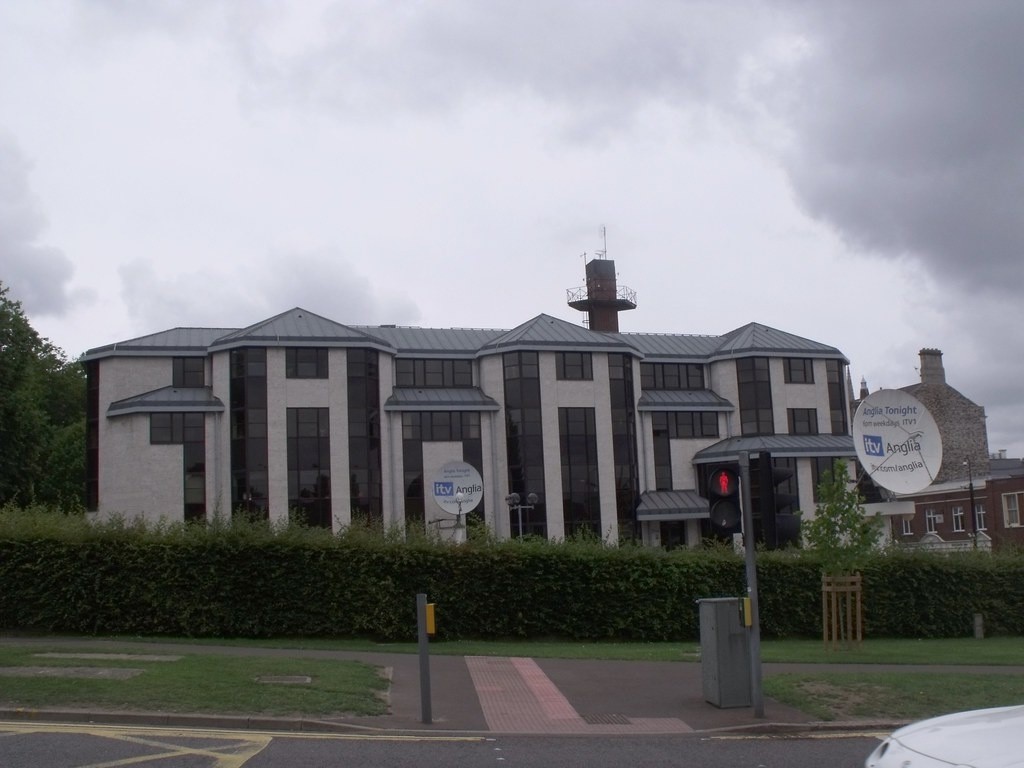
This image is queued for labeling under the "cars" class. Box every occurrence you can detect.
[866,704,1024,768]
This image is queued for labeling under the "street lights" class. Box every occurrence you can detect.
[963,454,977,547]
[505,492,538,540]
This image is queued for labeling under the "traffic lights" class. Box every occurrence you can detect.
[702,461,742,535]
[757,451,802,551]
